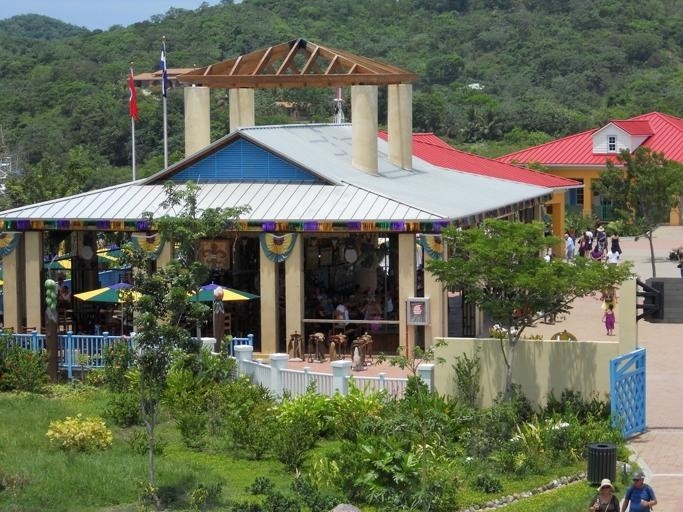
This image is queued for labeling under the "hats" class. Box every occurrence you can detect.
[598,478,615,493]
[632,472,644,480]
[597,226,604,232]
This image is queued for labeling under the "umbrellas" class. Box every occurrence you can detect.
[183,281,262,337]
[72,280,150,336]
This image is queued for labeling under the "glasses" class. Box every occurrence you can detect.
[602,486,609,489]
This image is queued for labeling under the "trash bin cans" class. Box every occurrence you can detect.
[587,443,617,487]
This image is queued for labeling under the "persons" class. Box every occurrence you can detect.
[620,471,657,512]
[599,304,614,336]
[304,267,396,338]
[585,479,620,512]
[564,233,574,263]
[598,286,617,316]
[57,284,71,308]
[577,215,622,266]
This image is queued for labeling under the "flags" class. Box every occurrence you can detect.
[159,38,171,98]
[128,72,140,122]
